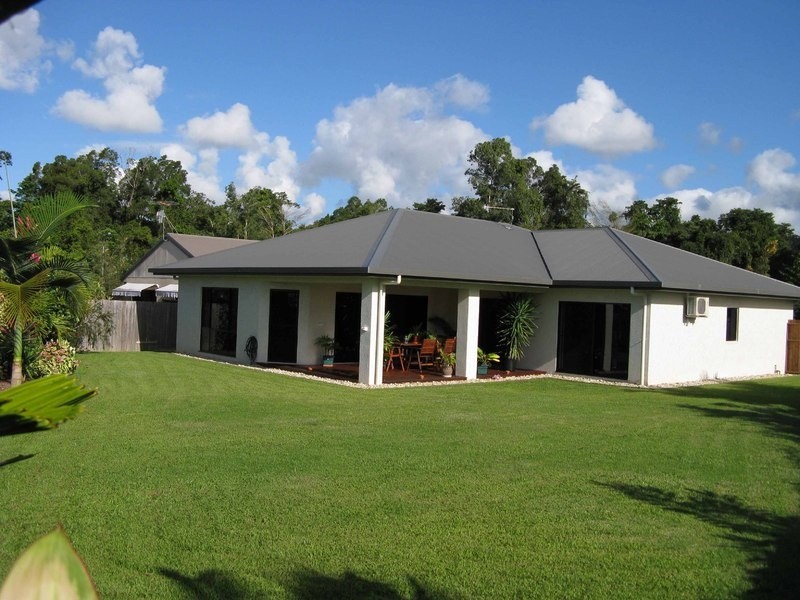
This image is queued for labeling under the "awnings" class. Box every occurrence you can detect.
[112,283,160,297]
[155,284,178,298]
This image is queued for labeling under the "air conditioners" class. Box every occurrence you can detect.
[685,295,710,318]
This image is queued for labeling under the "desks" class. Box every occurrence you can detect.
[392,342,422,370]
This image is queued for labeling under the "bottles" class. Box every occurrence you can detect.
[404,336,408,344]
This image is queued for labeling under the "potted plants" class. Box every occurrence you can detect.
[314,335,335,368]
[495,295,541,372]
[440,353,456,378]
[477,346,501,375]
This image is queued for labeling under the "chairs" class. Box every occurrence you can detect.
[387,345,406,372]
[415,338,437,374]
[438,339,456,359]
